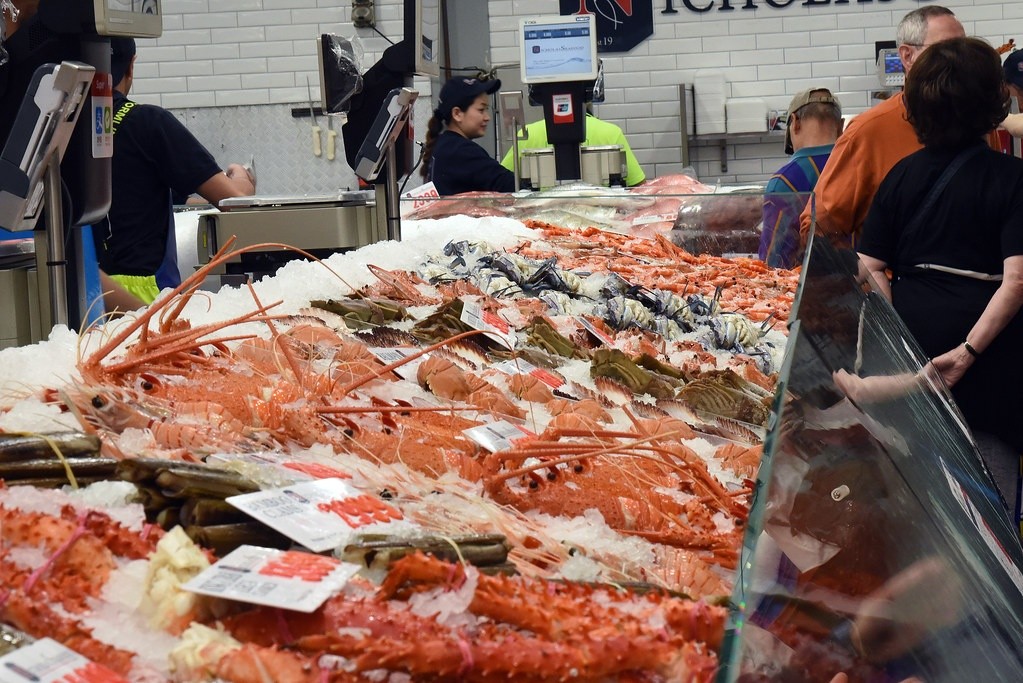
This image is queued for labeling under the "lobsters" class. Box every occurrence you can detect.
[59,230,764,575]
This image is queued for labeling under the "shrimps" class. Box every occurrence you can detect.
[357,217,802,390]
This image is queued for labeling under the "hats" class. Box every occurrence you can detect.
[784,87,842,155]
[1002,47,1023,88]
[438,76,501,120]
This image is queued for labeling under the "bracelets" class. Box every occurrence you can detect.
[964,340,978,358]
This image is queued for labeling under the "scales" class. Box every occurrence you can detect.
[193,86,420,275]
[0,60,97,351]
[517,13,627,191]
[72,0,163,228]
[875,47,906,93]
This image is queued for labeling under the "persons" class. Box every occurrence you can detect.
[90,36,258,320]
[799,5,1023,541]
[420,76,540,196]
[500,101,646,190]
[758,87,845,269]
[739,217,1022,683]
